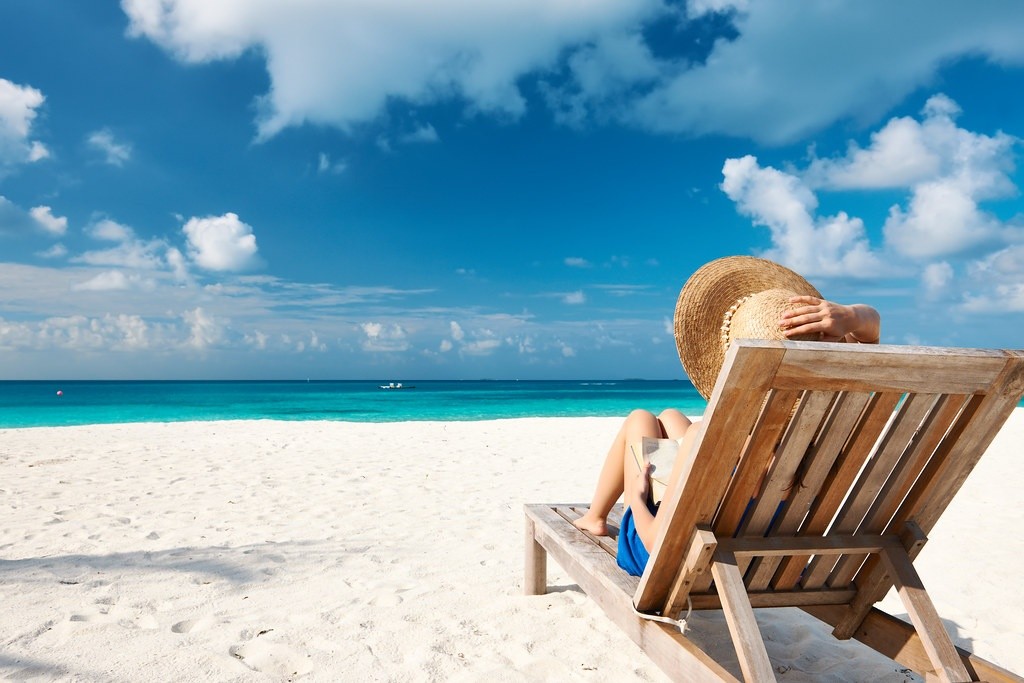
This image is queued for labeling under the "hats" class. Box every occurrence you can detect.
[674,255,849,446]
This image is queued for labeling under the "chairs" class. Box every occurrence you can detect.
[523,339,1024,682]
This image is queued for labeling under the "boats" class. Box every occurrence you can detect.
[377,384,416,389]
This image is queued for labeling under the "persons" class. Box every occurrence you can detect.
[570,295,881,578]
